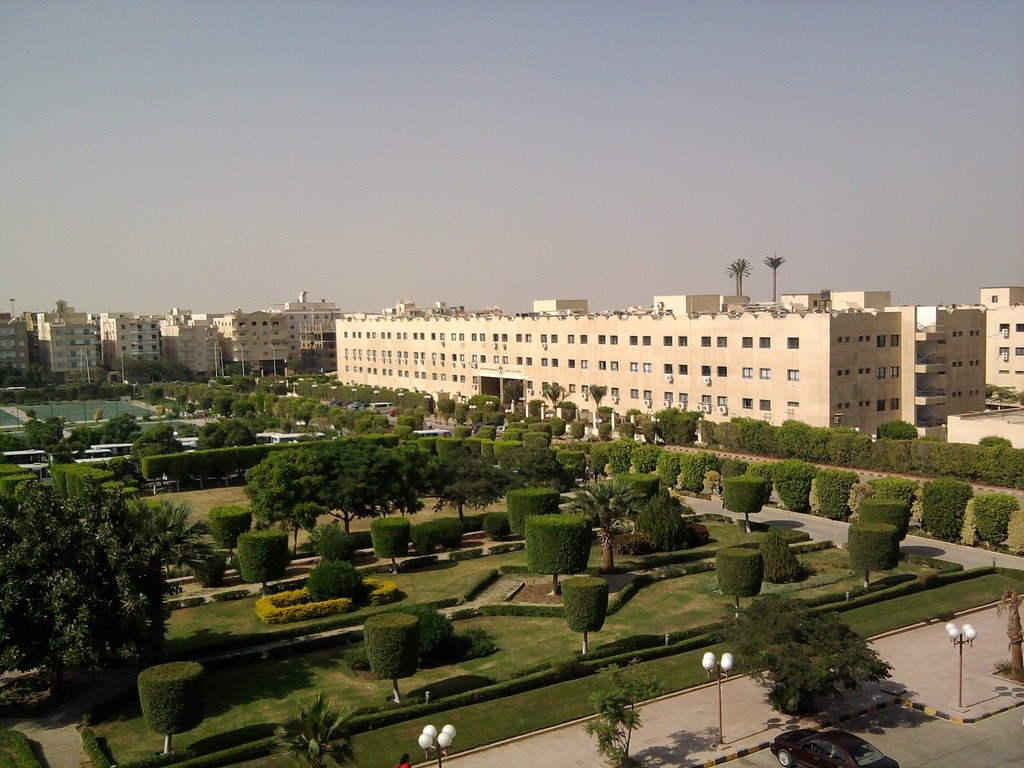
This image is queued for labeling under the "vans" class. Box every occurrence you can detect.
[496,426,508,436]
[370,403,395,414]
[7,386,26,390]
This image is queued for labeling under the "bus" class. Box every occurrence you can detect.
[3,450,51,471]
[72,449,116,462]
[414,428,453,438]
[91,444,135,459]
[271,431,326,442]
[176,437,198,452]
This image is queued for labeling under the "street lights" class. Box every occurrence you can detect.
[268,343,277,383]
[418,725,456,768]
[237,341,245,379]
[214,341,224,379]
[702,652,733,744]
[946,623,976,706]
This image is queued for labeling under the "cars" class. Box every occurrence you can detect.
[768,727,900,768]
[330,401,369,411]
